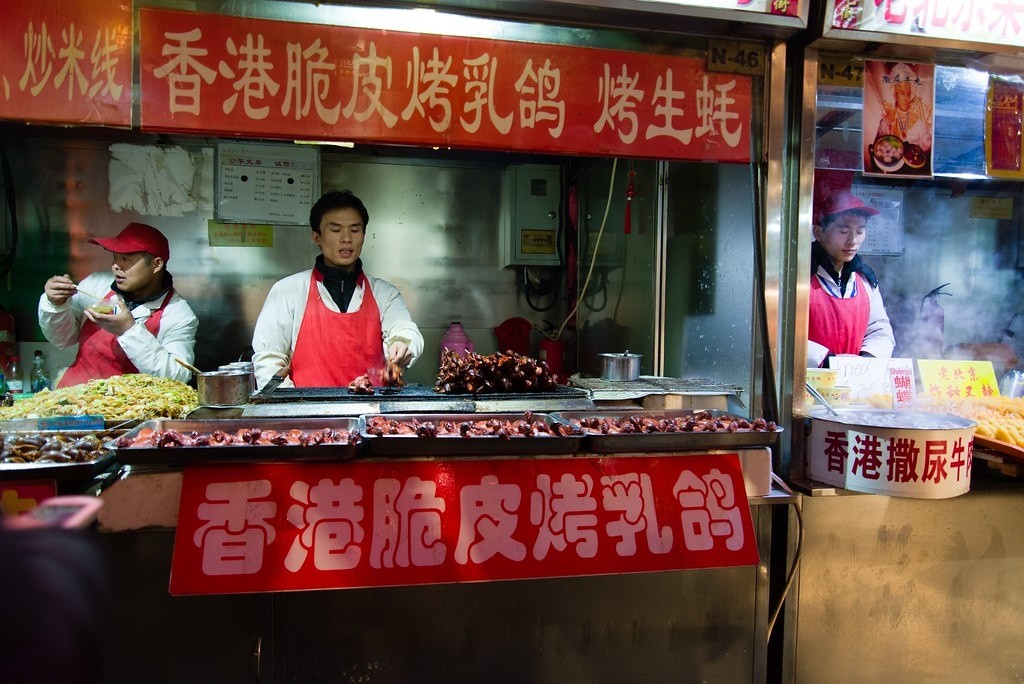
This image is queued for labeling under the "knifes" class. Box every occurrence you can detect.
[261,367,291,394]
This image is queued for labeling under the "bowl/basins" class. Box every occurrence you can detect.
[805,409,978,499]
[805,367,835,403]
[79,299,118,314]
[197,372,251,405]
[600,353,642,382]
[815,387,852,405]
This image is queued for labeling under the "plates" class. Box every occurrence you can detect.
[903,155,926,169]
[874,157,904,172]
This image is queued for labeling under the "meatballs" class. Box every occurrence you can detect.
[876,142,901,163]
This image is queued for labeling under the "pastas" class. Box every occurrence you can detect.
[0,373,199,419]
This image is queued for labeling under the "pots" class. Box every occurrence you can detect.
[869,135,909,165]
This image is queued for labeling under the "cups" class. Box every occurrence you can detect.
[230,362,255,394]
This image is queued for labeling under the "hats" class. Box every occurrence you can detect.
[812,190,881,224]
[884,61,920,91]
[86,222,169,264]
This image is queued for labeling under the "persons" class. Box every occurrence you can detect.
[251,189,424,386]
[806,188,896,368]
[38,223,198,388]
[875,80,933,152]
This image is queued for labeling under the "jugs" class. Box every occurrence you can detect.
[441,322,474,362]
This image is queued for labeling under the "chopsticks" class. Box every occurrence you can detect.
[73,283,103,299]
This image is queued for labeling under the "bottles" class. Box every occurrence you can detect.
[6,357,23,394]
[29,350,51,394]
[0,366,5,394]
